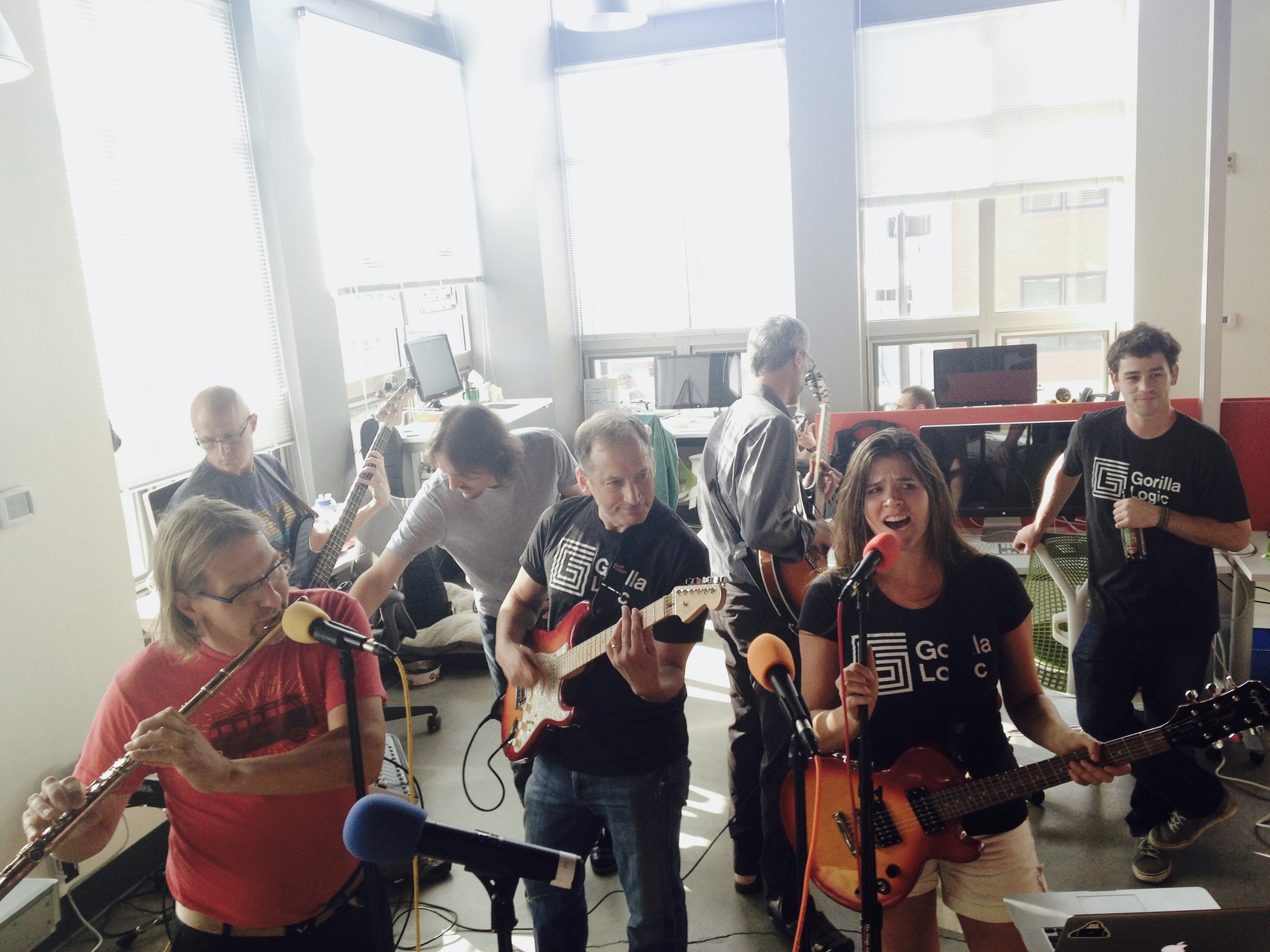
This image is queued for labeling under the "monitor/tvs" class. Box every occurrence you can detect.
[920,419,1087,543]
[404,334,464,411]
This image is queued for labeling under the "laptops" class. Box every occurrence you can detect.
[1002,886,1270,952]
[934,342,1037,408]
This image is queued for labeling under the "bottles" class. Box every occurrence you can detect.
[1118,489,1147,563]
[314,493,336,519]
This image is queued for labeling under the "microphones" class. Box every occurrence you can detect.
[839,532,900,603]
[281,602,397,660]
[747,633,821,757]
[343,794,585,890]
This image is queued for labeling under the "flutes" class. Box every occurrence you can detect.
[0,594,309,904]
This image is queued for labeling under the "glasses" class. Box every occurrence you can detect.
[193,416,249,449]
[802,351,816,374]
[196,544,293,606]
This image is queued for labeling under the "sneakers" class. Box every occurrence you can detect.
[1132,835,1173,883]
[1148,794,1241,850]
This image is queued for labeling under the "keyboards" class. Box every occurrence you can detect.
[968,542,1026,556]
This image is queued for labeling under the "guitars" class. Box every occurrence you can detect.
[288,374,419,590]
[778,676,1268,915]
[499,572,733,764]
[758,368,837,631]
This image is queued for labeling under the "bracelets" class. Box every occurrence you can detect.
[1156,507,1169,530]
[806,445,816,453]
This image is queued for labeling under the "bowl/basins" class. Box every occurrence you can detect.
[398,659,442,686]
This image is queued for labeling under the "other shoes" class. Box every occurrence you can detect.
[590,826,619,876]
[778,911,855,952]
[734,879,765,895]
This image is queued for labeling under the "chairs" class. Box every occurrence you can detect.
[632,415,700,529]
[367,589,438,733]
[1023,533,1091,698]
[1012,440,1068,508]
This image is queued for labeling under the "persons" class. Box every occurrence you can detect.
[346,400,616,874]
[797,428,1131,952]
[894,386,968,511]
[992,421,1086,506]
[697,314,856,952]
[1012,321,1252,882]
[164,387,390,589]
[495,408,711,951]
[796,420,817,458]
[22,491,393,952]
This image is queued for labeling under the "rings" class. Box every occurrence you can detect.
[611,644,621,647]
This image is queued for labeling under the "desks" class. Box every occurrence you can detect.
[632,407,729,447]
[962,528,1270,773]
[394,397,554,497]
[138,545,355,634]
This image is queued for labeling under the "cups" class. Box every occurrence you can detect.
[462,388,480,403]
